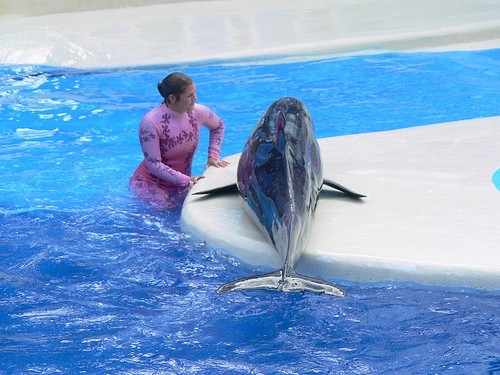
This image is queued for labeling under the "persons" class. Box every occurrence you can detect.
[127,72,232,210]
